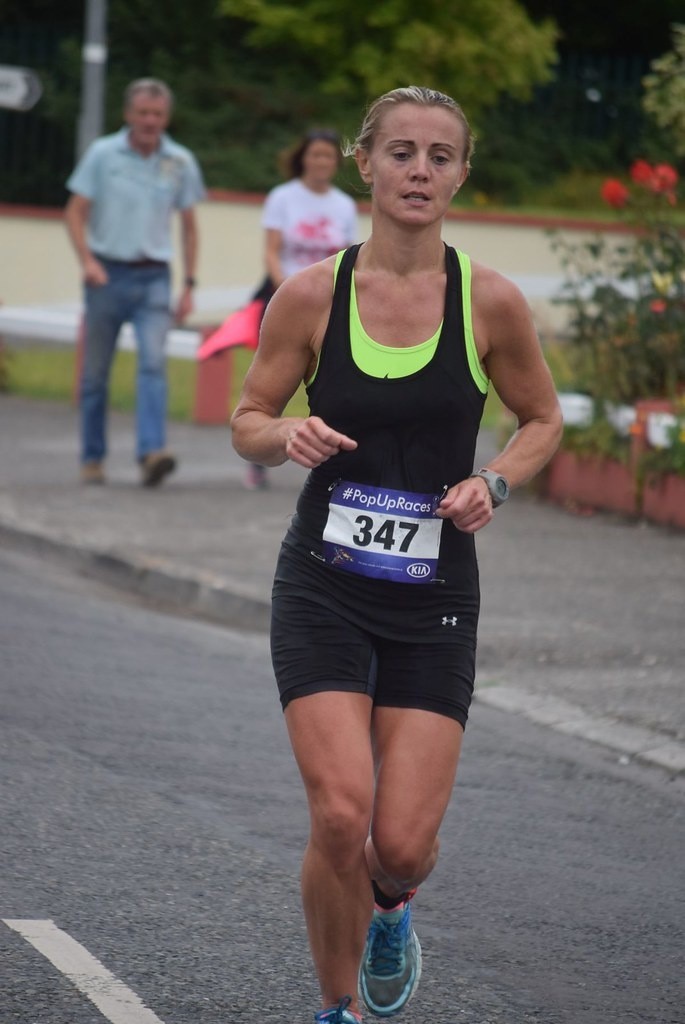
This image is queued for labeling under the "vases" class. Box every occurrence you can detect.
[543,392,684,527]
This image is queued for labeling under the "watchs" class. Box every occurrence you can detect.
[471,468,509,510]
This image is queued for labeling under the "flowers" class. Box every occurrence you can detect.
[548,157,685,471]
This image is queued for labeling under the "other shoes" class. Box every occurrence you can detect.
[140,452,176,487]
[79,460,103,483]
[243,459,266,490]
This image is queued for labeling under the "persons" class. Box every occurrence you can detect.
[241,132,361,492]
[232,87,563,1024]
[62,79,204,487]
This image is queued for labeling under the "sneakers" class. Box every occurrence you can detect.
[360,885,422,1017]
[314,995,361,1024]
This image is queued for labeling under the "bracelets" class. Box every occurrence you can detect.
[184,279,195,286]
